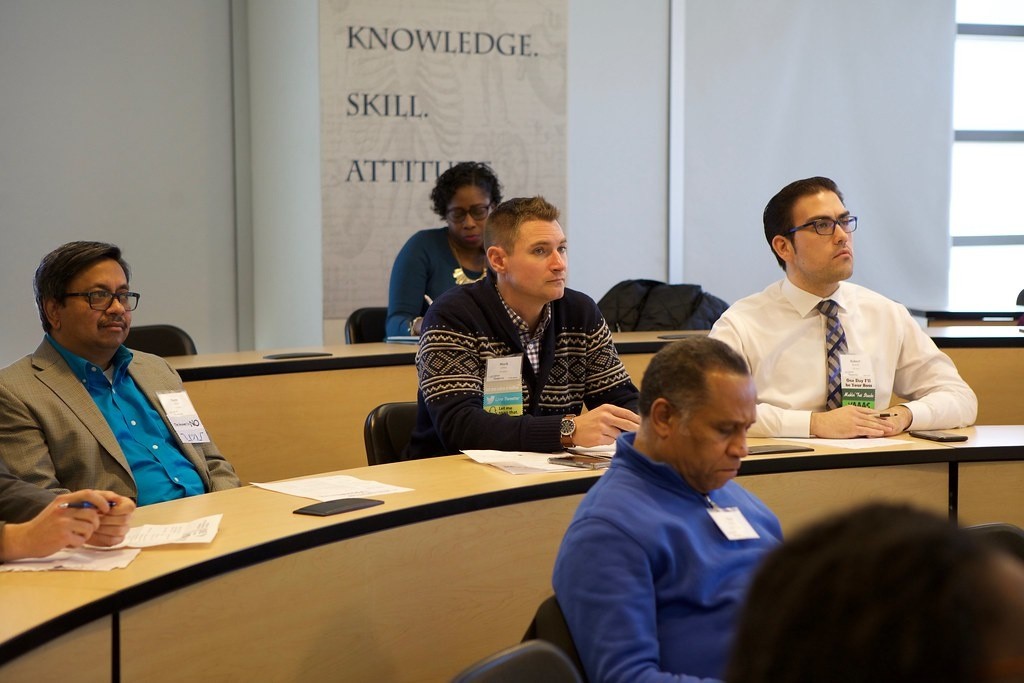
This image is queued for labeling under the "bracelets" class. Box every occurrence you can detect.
[410,317,422,335]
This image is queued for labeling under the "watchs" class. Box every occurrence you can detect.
[561,414,576,447]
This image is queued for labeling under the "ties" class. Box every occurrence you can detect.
[817,300,848,411]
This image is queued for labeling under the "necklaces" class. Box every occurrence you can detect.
[452,246,488,285]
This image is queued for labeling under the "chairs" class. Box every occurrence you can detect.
[120,325,197,358]
[365,402,420,466]
[344,307,388,345]
[451,594,590,683]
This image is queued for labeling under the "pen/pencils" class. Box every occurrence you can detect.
[58,501,115,509]
[870,414,898,417]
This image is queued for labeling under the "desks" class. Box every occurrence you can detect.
[0,316,1023,683]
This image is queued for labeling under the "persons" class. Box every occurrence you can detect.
[709,176,978,439]
[385,161,503,337]
[553,335,785,682]
[729,504,1024,683]
[0,460,135,561]
[0,240,239,506]
[416,196,640,460]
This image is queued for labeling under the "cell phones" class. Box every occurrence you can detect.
[909,431,968,442]
[548,455,612,470]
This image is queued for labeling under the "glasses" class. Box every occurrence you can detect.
[782,216,858,235]
[55,290,140,311]
[443,199,494,223]
